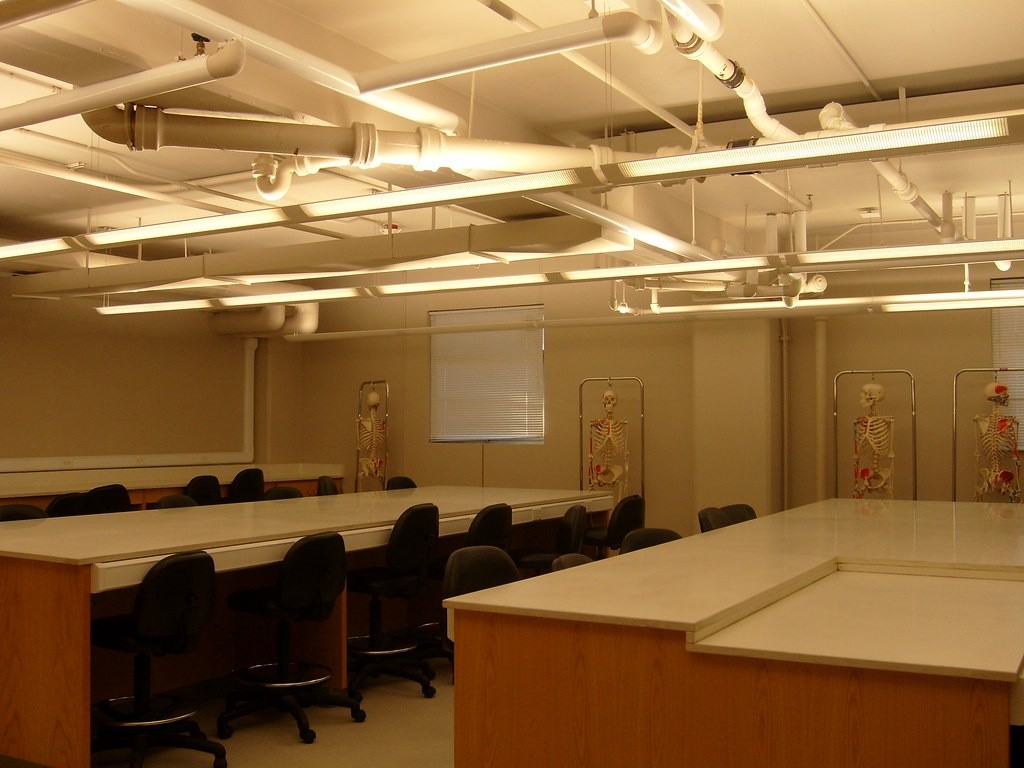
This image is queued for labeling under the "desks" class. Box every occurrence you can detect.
[0,486,614,767]
[0,464,347,512]
[443,498,1024,768]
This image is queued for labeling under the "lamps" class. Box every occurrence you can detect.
[0,109,1023,314]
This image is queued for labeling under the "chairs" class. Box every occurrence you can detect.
[89,549,226,767]
[416,495,756,684]
[216,532,367,743]
[0,472,419,522]
[348,502,456,698]
